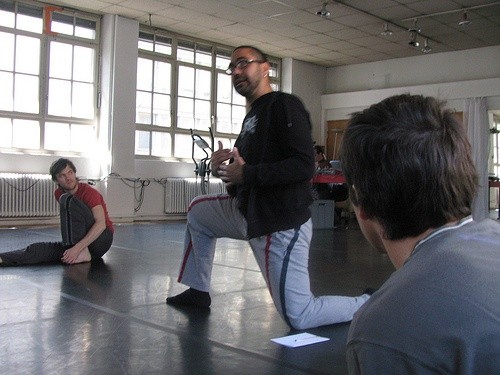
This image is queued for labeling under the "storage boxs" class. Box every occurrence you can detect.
[310,199,334,229]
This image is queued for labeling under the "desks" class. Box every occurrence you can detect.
[488,180,500,220]
[309,175,350,230]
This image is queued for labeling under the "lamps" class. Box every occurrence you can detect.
[315,0,331,16]
[380,22,393,36]
[422,37,432,52]
[457,9,472,27]
[407,19,420,46]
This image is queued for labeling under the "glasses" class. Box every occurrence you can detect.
[225,60,266,75]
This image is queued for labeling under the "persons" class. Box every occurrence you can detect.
[0,158,114,268]
[166,45,375,332]
[344,94,500,375]
[312,144,332,199]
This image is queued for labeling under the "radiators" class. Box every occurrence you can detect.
[165,178,226,214]
[0,171,60,217]
